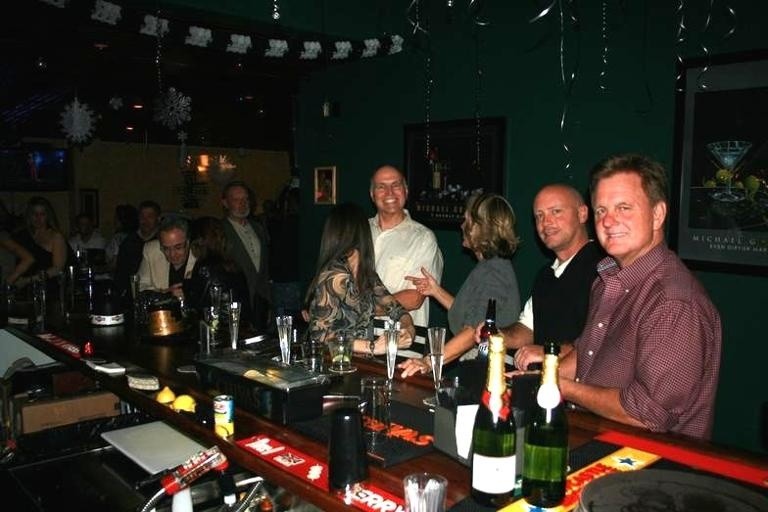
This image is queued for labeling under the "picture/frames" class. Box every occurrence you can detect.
[311,164,338,205]
[403,114,508,234]
[665,49,768,277]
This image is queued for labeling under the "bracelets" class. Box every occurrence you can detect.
[369,339,375,356]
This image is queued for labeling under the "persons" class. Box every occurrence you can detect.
[136,216,251,327]
[366,161,445,329]
[67,200,162,300]
[398,189,521,379]
[475,183,604,376]
[221,182,323,327]
[302,198,418,354]
[1,197,69,297]
[536,150,723,444]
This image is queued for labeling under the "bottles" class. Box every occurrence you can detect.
[467,335,516,509]
[521,341,569,509]
[413,201,464,219]
[475,296,498,357]
[213,458,292,512]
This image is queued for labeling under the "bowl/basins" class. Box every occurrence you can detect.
[577,469,768,511]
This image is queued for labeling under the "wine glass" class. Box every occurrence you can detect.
[704,139,752,203]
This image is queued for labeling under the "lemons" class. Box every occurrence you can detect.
[173,394,196,412]
[156,385,175,404]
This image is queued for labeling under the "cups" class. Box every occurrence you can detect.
[432,160,450,190]
[300,338,325,374]
[325,333,352,369]
[381,330,404,383]
[359,377,392,447]
[327,406,366,491]
[274,314,293,365]
[25,262,140,330]
[201,286,240,350]
[400,471,448,511]
[426,324,446,401]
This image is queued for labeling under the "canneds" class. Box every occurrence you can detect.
[212,393,236,439]
